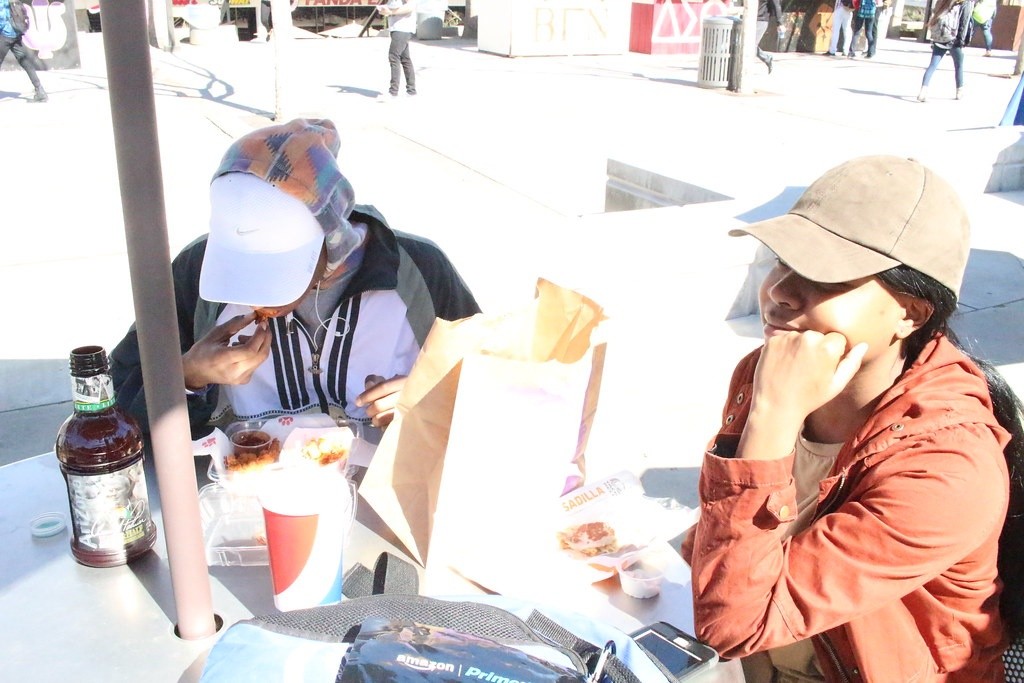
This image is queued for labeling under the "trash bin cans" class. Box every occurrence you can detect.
[697,16,740,89]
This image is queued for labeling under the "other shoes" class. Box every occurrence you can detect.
[865,51,874,60]
[376,92,398,103]
[847,53,855,57]
[983,50,991,57]
[766,56,774,74]
[27,93,48,104]
[822,51,836,57]
[399,91,417,101]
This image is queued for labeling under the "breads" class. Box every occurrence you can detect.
[570,522,614,553]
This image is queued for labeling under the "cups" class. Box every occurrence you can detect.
[256,472,350,612]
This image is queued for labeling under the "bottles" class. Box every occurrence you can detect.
[56,345,157,568]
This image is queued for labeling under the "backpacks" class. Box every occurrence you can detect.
[8,0,30,34]
[930,1,970,44]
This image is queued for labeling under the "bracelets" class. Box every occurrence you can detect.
[389,10,392,16]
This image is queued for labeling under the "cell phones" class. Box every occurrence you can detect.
[627,621,719,682]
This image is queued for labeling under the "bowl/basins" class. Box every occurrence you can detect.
[230,429,271,457]
[207,418,364,482]
[612,549,666,598]
[197,480,358,566]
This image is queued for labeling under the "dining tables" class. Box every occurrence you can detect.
[1,417,747,682]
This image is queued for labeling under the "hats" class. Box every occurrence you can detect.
[198,173,325,306]
[728,157,971,309]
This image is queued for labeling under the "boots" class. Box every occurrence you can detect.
[917,86,926,102]
[956,87,963,101]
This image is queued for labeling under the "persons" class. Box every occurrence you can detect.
[0,0,48,102]
[917,0,996,102]
[681,154,1023,683]
[261,0,296,41]
[822,0,891,59]
[379,0,417,102]
[757,0,786,74]
[106,120,483,440]
